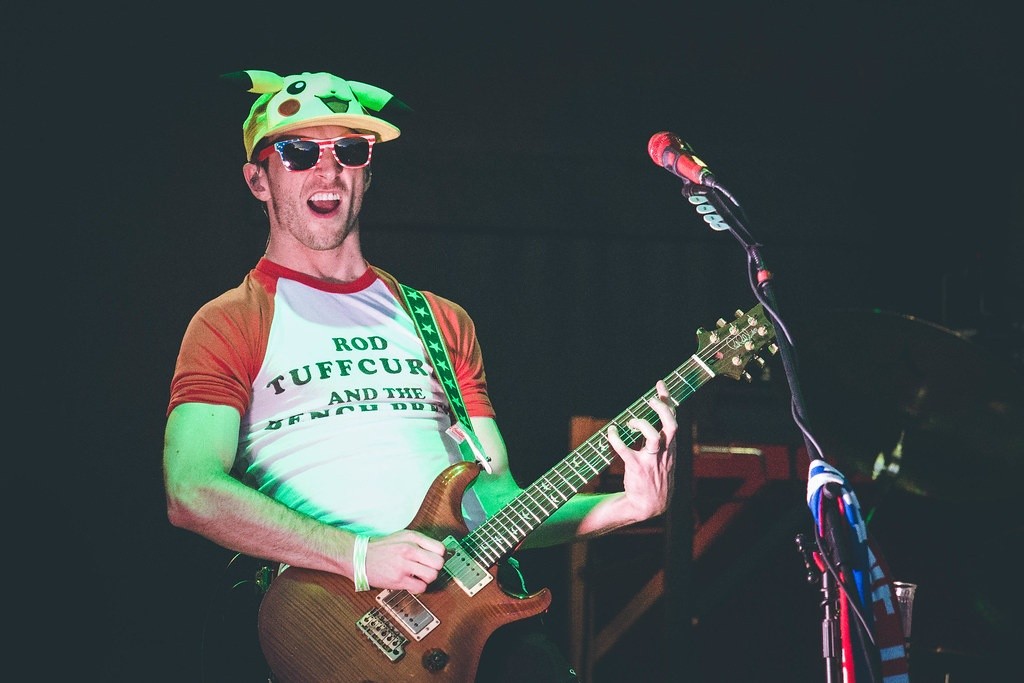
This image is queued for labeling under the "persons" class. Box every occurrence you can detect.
[162,69,680,683]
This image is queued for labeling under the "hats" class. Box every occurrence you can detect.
[242,70,400,164]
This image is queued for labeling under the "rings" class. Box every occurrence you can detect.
[644,446,661,455]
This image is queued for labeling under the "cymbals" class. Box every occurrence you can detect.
[792,306,1004,437]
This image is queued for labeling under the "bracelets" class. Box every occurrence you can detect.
[353,533,371,592]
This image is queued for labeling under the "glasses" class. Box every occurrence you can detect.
[258,133,377,171]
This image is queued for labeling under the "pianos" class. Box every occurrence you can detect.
[571,415,842,478]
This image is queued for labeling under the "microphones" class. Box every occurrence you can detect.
[648,131,716,187]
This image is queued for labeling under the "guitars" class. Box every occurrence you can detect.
[256,300,783,683]
